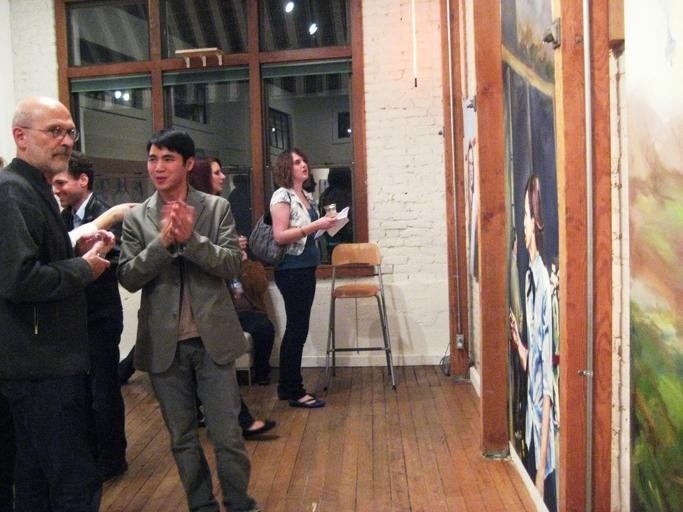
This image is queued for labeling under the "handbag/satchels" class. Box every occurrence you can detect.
[249,208,291,264]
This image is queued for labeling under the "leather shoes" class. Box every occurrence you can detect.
[289,393,325,408]
[243,419,277,436]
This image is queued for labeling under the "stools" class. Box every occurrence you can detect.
[229,331,254,391]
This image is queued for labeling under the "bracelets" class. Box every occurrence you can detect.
[300,227,307,239]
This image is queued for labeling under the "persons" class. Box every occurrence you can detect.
[53,152,129,482]
[508,175,557,512]
[118,128,252,509]
[184,157,275,439]
[268,149,337,409]
[228,251,275,385]
[462,139,480,285]
[0,96,112,511]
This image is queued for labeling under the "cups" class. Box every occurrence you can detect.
[323,203,336,219]
[87,235,108,258]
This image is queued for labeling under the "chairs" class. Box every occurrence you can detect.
[324,243,397,391]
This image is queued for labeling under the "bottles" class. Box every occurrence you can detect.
[230,276,244,298]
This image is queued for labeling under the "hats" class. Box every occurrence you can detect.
[239,259,268,310]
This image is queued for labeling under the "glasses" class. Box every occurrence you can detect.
[20,125,80,141]
